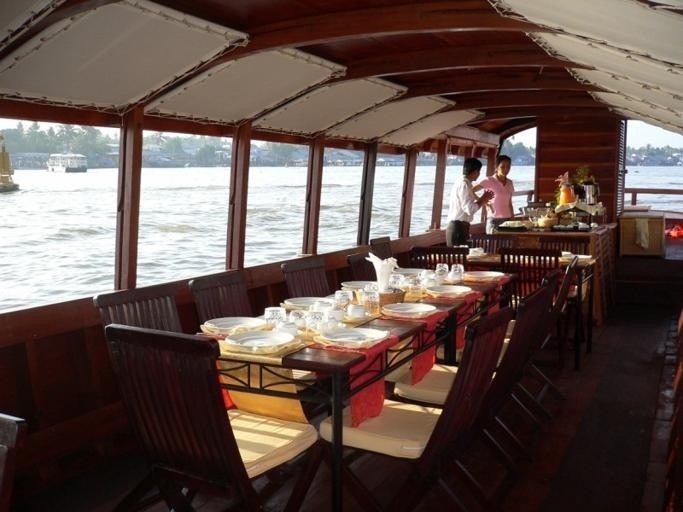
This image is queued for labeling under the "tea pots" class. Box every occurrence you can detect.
[559,182,576,203]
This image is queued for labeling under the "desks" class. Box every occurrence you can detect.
[493,222,618,329]
[413,246,595,350]
[197,268,508,507]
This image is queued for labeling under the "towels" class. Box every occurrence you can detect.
[635,218,649,249]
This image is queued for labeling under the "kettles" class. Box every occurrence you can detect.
[583,180,599,205]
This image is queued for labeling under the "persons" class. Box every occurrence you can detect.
[472,155,514,235]
[445,157,495,248]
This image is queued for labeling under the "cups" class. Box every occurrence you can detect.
[390,264,464,298]
[335,290,378,319]
[264,307,323,335]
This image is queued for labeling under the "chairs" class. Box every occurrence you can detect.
[539,234,595,352]
[295,309,516,511]
[0,412,27,512]
[372,238,393,261]
[347,249,380,283]
[105,322,344,511]
[409,245,431,268]
[98,287,181,335]
[471,233,516,253]
[498,243,566,300]
[188,270,255,322]
[396,256,579,471]
[281,254,331,299]
[428,245,469,272]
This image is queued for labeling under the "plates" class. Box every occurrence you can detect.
[205,317,264,330]
[322,328,387,343]
[464,270,502,278]
[428,285,471,295]
[226,331,294,349]
[383,302,434,314]
[341,281,378,288]
[284,297,332,307]
